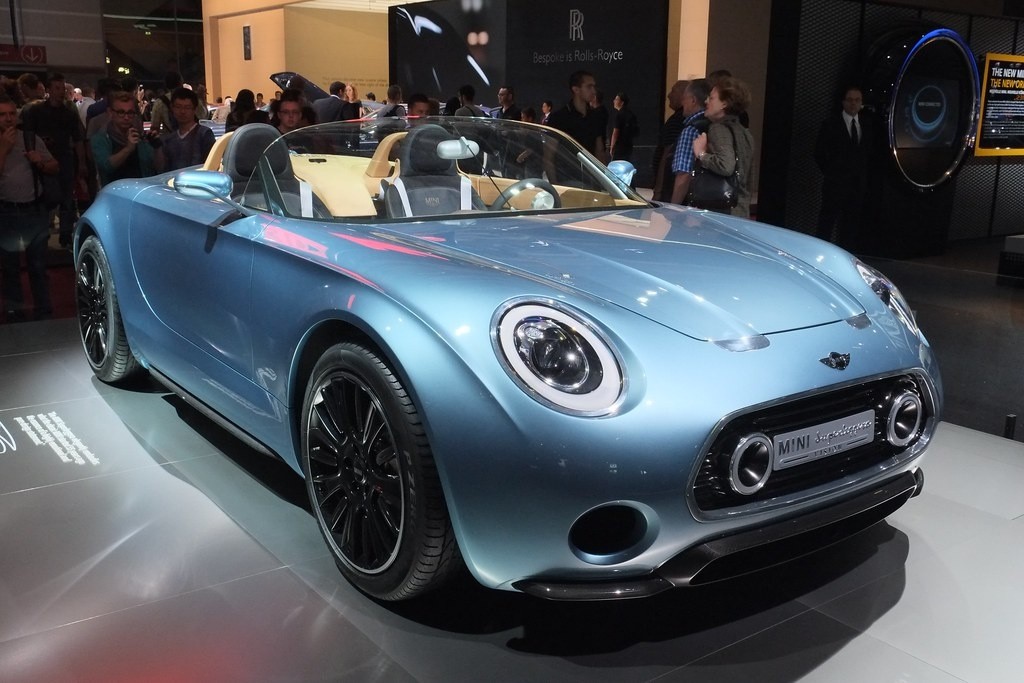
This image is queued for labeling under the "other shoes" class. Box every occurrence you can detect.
[33,300,54,320]
[58,232,73,247]
[3,299,24,322]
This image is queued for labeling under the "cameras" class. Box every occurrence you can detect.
[133,131,149,140]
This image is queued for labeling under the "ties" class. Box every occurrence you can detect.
[850,119,858,150]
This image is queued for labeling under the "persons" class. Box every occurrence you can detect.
[539,100,551,129]
[811,87,875,253]
[161,88,215,170]
[224,87,270,132]
[0,92,60,319]
[588,89,608,141]
[495,85,523,179]
[0,71,387,234]
[375,88,407,144]
[19,71,87,253]
[406,90,464,134]
[451,85,485,176]
[651,67,756,220]
[88,89,165,190]
[609,92,634,162]
[297,106,332,144]
[273,88,314,153]
[516,108,544,179]
[542,70,605,190]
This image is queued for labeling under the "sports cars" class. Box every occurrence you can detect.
[74,70,943,603]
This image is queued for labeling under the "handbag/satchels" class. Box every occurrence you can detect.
[39,177,64,209]
[682,121,739,215]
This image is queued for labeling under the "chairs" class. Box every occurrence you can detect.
[385,124,488,219]
[222,122,334,218]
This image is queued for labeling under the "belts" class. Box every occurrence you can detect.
[2,201,33,208]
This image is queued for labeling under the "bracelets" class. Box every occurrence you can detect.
[698,152,706,159]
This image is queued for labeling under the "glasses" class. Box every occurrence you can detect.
[110,107,134,115]
[278,108,301,116]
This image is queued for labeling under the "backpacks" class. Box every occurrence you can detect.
[375,105,403,142]
